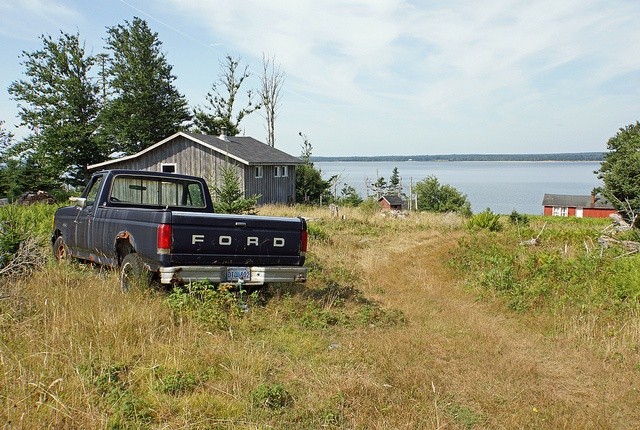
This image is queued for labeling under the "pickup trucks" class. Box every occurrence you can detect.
[51,169,308,297]
